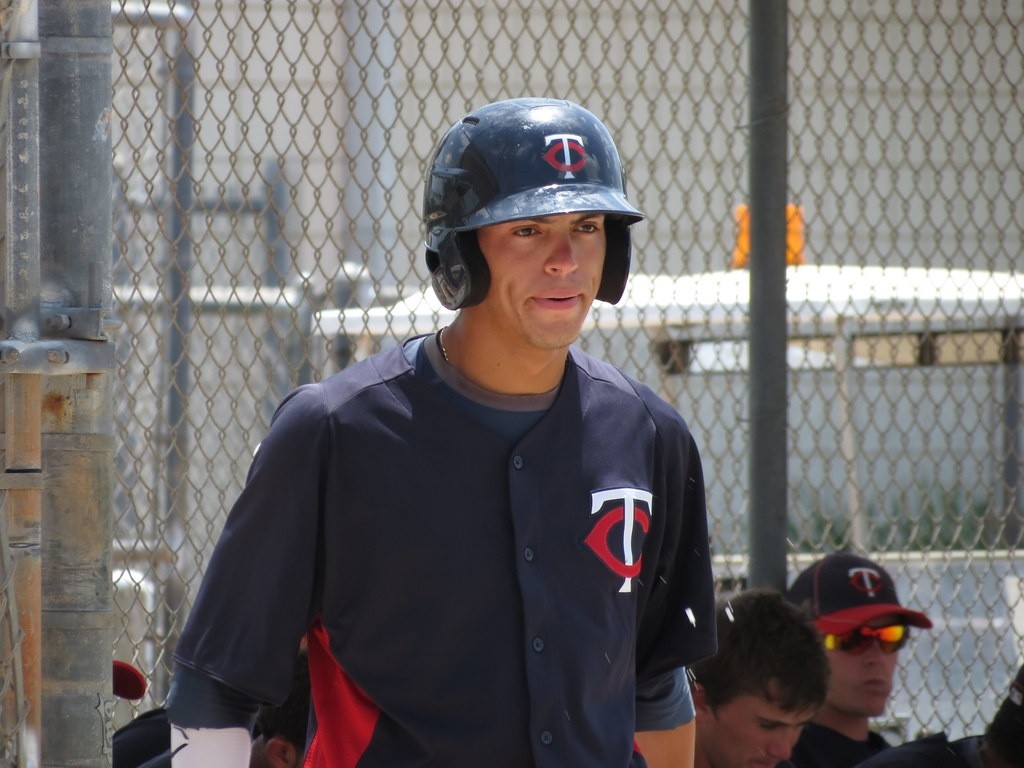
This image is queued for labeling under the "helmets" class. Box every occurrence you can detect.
[423,98,645,310]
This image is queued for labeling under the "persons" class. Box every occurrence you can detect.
[630,550,1024,768]
[164,96,715,767]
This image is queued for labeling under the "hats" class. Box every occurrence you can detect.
[784,553,933,637]
[113,659,147,700]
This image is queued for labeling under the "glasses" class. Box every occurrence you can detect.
[821,623,909,654]
